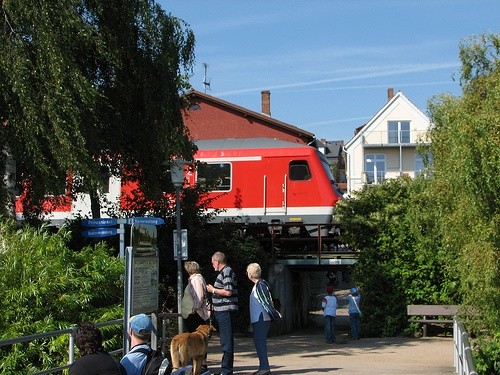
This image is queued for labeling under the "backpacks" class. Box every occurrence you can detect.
[132,348,172,375]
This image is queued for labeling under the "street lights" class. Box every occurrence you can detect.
[171,166,184,338]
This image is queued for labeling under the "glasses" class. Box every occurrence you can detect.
[246,271,248,275]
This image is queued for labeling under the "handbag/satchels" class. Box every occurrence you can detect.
[272,298,281,320]
[359,311,364,321]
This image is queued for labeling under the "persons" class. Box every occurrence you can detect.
[346,287,362,341]
[206,252,241,375]
[67,323,128,375]
[181,261,210,370]
[247,263,283,375]
[120,313,164,375]
[321,287,337,344]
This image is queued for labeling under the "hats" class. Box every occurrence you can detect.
[129,313,152,335]
[350,287,358,293]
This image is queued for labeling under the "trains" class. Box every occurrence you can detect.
[15,138,339,235]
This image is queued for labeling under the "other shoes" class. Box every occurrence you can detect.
[350,335,359,340]
[214,368,223,375]
[326,338,336,344]
[199,364,210,375]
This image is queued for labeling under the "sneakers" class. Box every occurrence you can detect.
[255,369,270,375]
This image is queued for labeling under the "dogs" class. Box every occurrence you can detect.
[170,325,216,375]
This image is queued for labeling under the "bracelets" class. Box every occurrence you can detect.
[213,288,215,294]
